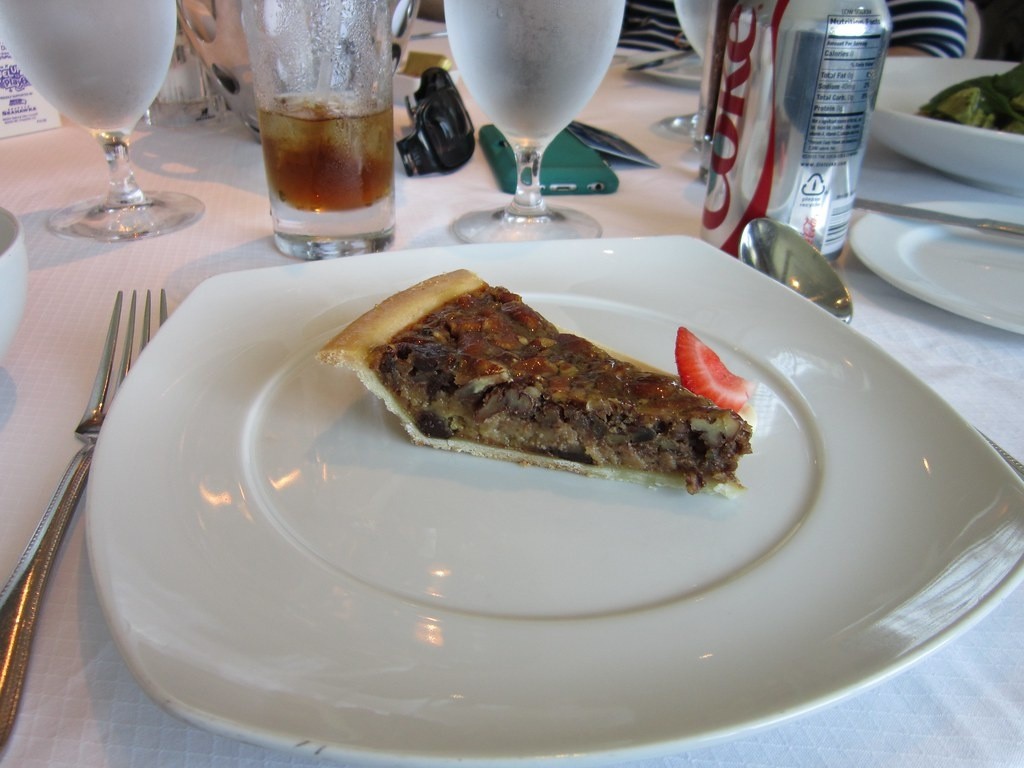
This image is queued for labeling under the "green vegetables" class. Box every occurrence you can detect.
[919,61,1024,138]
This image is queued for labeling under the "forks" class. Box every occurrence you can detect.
[0,289,166,753]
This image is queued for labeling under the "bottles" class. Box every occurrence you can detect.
[699,1,739,184]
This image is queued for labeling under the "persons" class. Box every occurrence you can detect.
[617,0,966,59]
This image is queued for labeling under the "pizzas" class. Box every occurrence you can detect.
[317,269,754,499]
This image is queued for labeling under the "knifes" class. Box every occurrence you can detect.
[852,197,1023,240]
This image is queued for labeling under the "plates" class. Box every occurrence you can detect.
[849,211,1023,335]
[83,235,1023,762]
[631,49,707,87]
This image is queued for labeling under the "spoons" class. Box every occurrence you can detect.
[736,219,1024,483]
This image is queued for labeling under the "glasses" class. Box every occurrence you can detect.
[396,67,475,176]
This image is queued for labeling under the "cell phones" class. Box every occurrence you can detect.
[479,124,620,196]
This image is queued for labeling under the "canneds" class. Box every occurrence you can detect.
[700,0,891,273]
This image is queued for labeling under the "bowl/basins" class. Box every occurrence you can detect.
[177,0,420,143]
[869,56,1024,198]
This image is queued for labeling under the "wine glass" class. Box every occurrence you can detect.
[660,0,724,142]
[1,0,205,242]
[444,0,626,241]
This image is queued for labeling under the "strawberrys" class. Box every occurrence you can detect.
[675,325,753,416]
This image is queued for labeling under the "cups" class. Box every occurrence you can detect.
[0,208,28,366]
[241,1,396,260]
